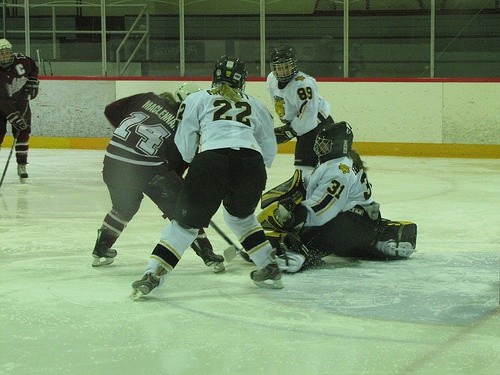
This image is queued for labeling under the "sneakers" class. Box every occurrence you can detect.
[129,271,158,304]
[277,251,306,273]
[92,229,118,267]
[17,164,29,181]
[374,238,418,258]
[202,247,228,273]
[250,263,284,290]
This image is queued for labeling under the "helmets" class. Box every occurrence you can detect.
[0,39,13,65]
[270,45,300,85]
[314,121,354,163]
[177,82,202,103]
[212,54,249,88]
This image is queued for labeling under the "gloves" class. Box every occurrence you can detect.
[274,125,297,145]
[7,111,30,133]
[25,78,40,100]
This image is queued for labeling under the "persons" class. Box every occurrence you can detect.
[92,82,225,273]
[131,55,283,299]
[0,39,40,183]
[266,45,334,184]
[277,121,416,274]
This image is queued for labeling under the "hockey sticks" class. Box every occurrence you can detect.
[209,220,256,265]
[0,93,30,189]
